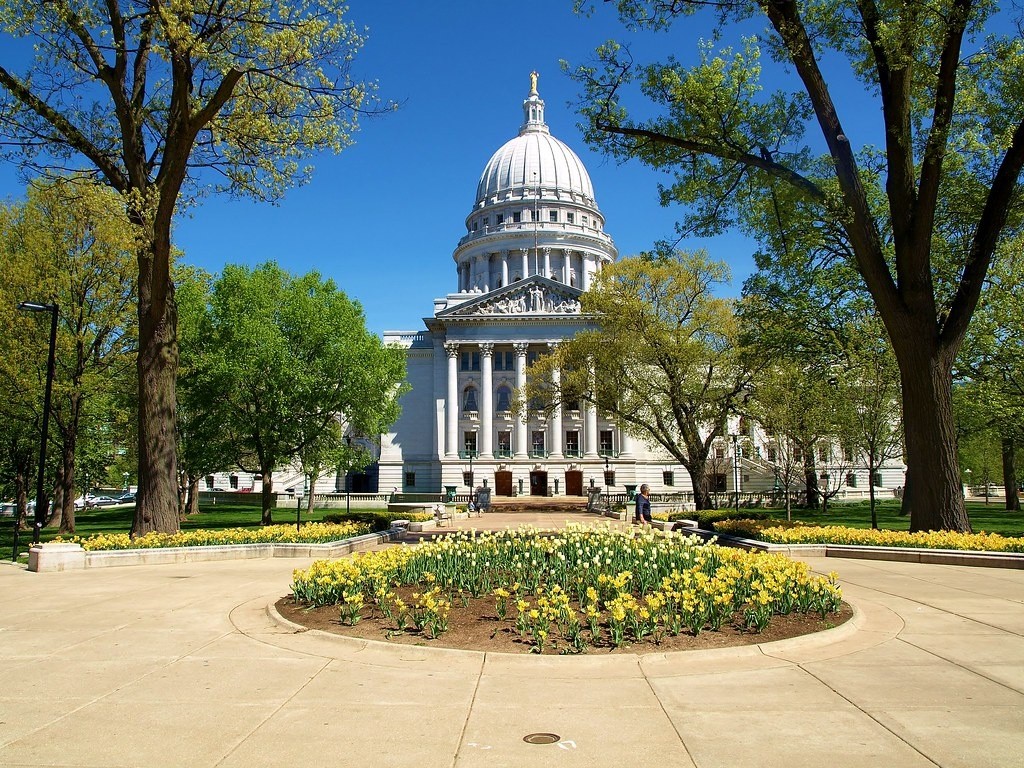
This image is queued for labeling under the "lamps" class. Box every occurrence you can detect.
[570,462,578,470]
[499,463,506,470]
[534,463,542,471]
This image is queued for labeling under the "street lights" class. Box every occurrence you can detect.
[16,301,60,545]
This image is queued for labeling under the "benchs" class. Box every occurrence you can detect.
[435,507,453,527]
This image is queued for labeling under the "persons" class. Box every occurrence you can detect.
[470,286,582,315]
[394,486,398,493]
[27,504,32,513]
[82,501,91,511]
[799,497,806,504]
[468,500,484,518]
[898,486,905,496]
[636,484,653,533]
[530,71,539,91]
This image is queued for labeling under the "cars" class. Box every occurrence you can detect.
[207,488,225,492]
[234,487,252,493]
[118,492,138,504]
[73,495,96,509]
[86,496,123,507]
